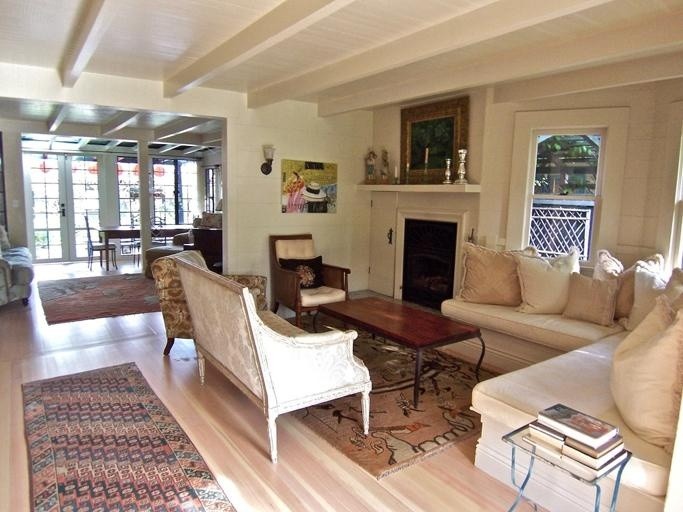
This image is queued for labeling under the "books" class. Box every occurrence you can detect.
[522,403,628,478]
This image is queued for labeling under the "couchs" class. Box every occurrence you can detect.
[440,248,683,512]
[0,226,35,310]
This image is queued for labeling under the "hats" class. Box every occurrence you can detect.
[304,182,326,199]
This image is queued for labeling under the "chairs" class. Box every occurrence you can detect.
[150,249,268,357]
[268,232,352,326]
[79,196,224,279]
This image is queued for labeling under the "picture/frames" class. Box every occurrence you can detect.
[396,94,471,184]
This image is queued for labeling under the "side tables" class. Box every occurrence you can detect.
[501,417,633,512]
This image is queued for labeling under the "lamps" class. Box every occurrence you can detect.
[261,146,275,176]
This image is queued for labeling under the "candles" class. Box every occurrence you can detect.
[424,149,429,163]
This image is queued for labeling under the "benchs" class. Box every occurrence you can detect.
[174,255,372,462]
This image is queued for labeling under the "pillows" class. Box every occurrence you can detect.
[592,249,665,320]
[512,249,581,314]
[610,295,681,453]
[617,266,669,331]
[458,247,541,306]
[279,255,324,291]
[562,272,618,328]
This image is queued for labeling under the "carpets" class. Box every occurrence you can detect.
[19,361,235,511]
[36,275,163,325]
[294,330,496,484]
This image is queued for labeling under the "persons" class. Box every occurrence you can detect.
[366,150,377,181]
[284,171,328,213]
